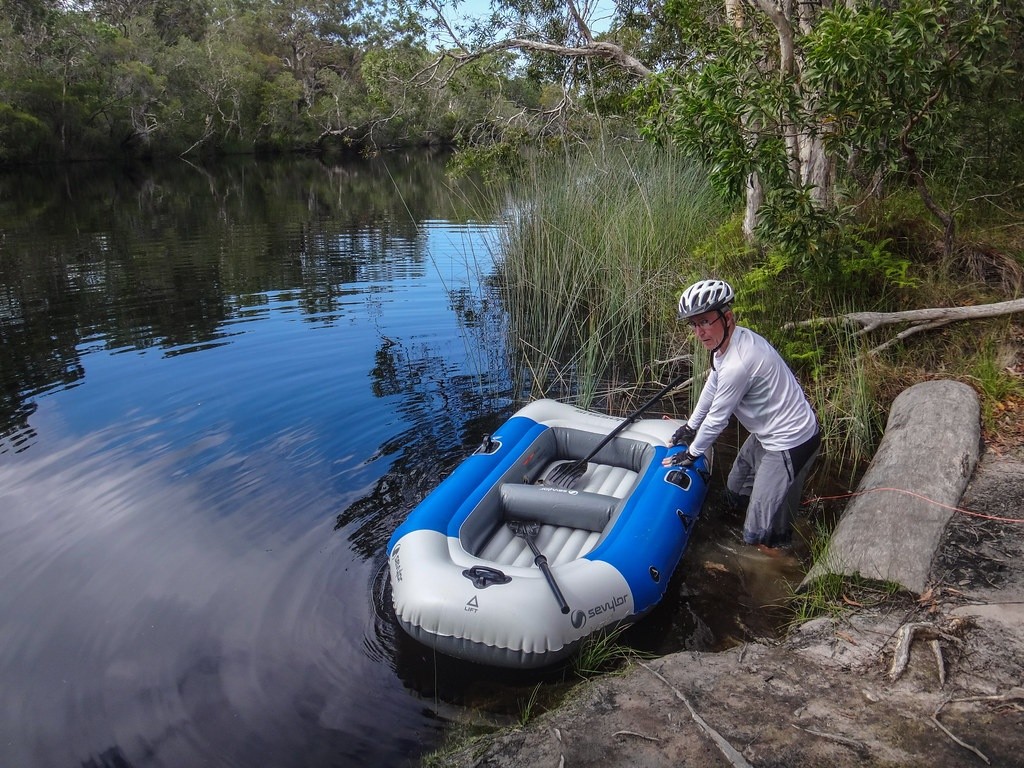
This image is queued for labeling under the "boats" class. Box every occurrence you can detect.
[382,400,715,667]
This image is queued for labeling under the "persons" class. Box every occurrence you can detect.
[662,278,822,548]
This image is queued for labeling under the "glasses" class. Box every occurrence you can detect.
[685,310,727,330]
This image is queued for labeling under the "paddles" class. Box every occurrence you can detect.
[544,372,687,490]
[508,517,570,615]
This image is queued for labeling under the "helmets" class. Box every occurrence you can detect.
[675,280,734,320]
